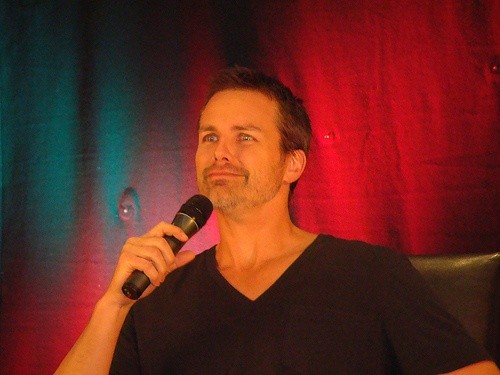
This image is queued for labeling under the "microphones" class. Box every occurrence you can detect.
[121,194,214,300]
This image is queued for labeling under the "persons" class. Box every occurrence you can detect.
[54,66,500,374]
